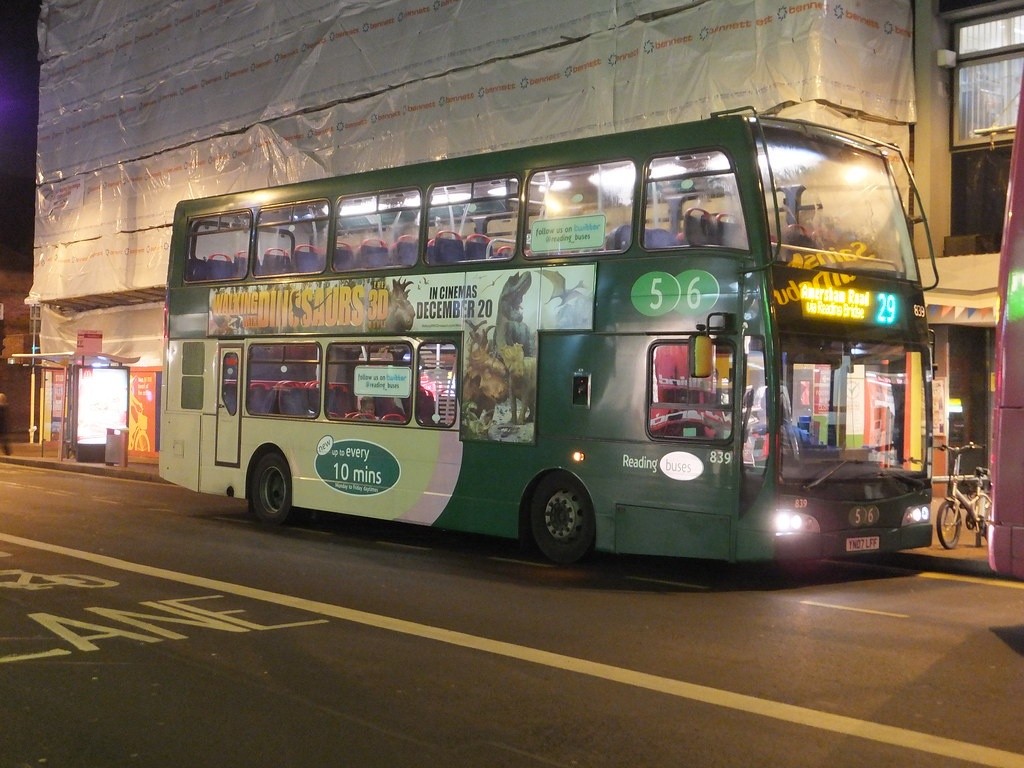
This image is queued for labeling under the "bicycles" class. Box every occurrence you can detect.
[987,65,1024,578]
[936,442,990,549]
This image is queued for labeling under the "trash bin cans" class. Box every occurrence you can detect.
[104,426,130,468]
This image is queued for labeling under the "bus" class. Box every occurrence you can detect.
[159,106,938,572]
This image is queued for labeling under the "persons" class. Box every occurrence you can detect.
[745,426,811,448]
[360,397,379,420]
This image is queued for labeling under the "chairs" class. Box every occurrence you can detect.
[225,379,437,425]
[202,207,816,280]
